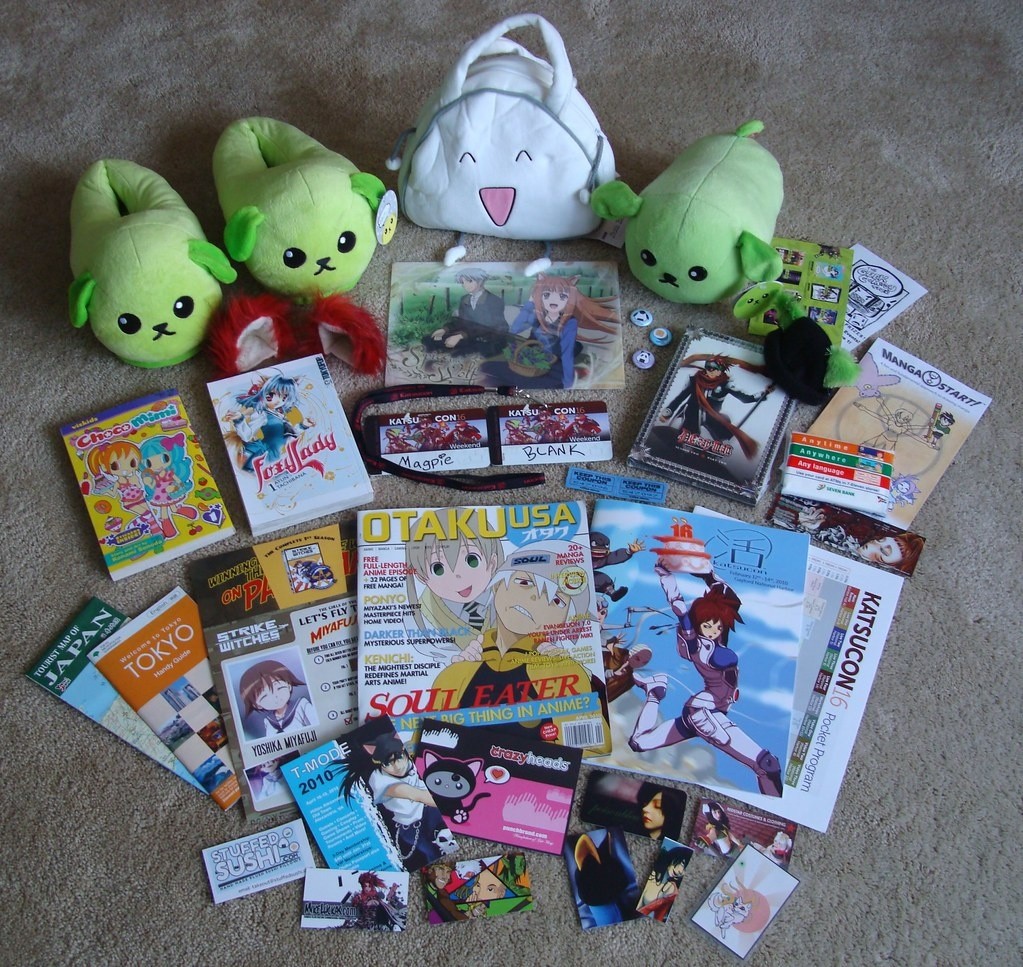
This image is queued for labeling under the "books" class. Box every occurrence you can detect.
[206,353,374,537]
[58,387,236,581]
[778,336,993,532]
[23,501,906,874]
[627,323,797,506]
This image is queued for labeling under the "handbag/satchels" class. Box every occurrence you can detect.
[395,11,616,241]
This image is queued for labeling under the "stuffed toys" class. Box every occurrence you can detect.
[67,157,236,369]
[385,13,621,277]
[730,278,861,405]
[211,118,398,302]
[590,121,783,305]
[203,288,385,381]
